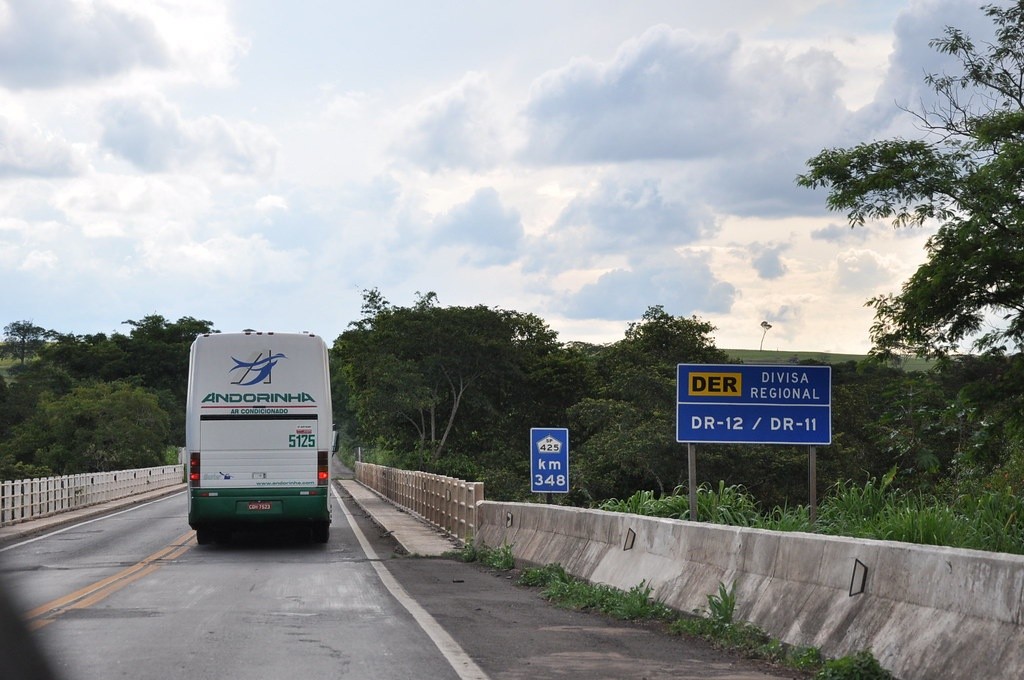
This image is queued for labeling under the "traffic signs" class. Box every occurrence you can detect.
[677,363,832,445]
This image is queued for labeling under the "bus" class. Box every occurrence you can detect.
[184,327,339,544]
